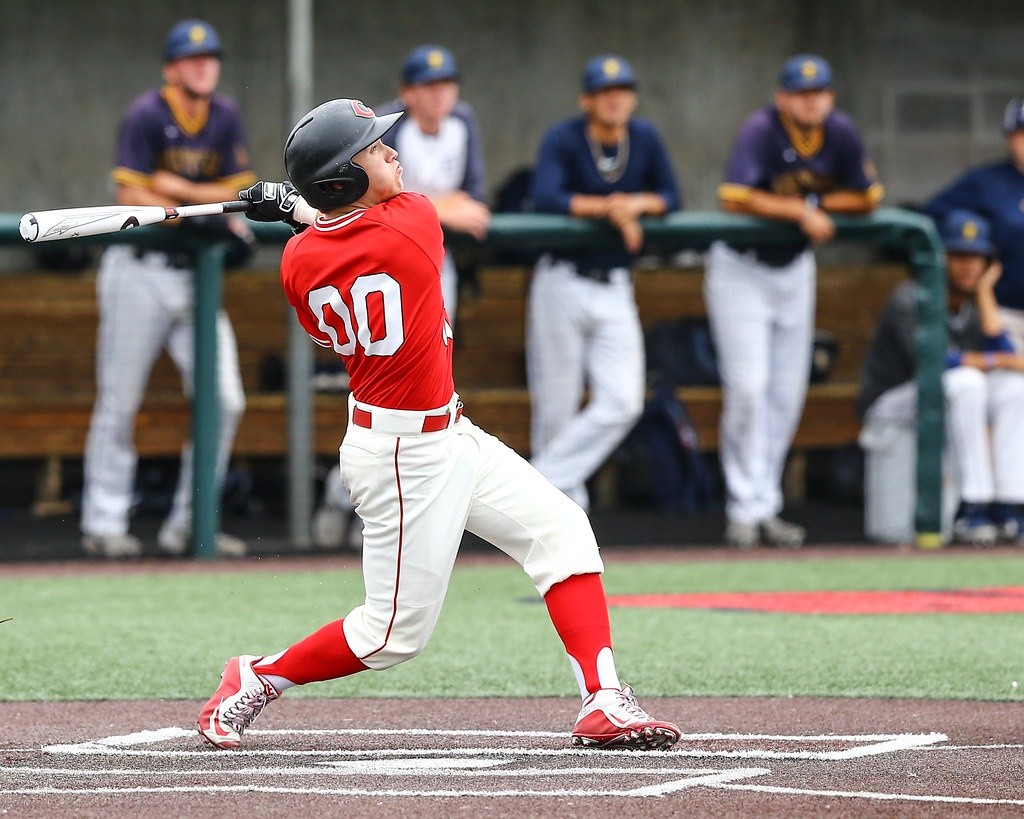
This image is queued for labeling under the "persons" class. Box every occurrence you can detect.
[198,99,681,753]
[701,53,883,548]
[854,94,1024,547]
[313,44,490,552]
[83,16,259,558]
[527,55,683,511]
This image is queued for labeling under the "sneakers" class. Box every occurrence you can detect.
[572,681,681,751]
[197,653,283,749]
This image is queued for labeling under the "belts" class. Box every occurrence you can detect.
[351,404,464,434]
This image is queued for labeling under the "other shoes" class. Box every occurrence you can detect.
[724,522,761,551]
[159,527,251,557]
[81,533,143,555]
[951,503,1022,550]
[760,518,807,546]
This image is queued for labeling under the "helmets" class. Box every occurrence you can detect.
[282,99,407,214]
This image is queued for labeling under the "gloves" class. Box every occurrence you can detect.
[237,181,310,235]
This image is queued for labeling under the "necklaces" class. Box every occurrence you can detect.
[585,123,631,184]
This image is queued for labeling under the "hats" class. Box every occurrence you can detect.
[401,42,457,87]
[938,208,994,255]
[581,53,637,95]
[162,18,225,62]
[777,52,834,92]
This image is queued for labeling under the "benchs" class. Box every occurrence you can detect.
[0,255,909,517]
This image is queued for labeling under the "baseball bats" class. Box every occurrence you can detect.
[16,198,253,243]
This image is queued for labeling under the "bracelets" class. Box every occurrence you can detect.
[800,190,824,209]
